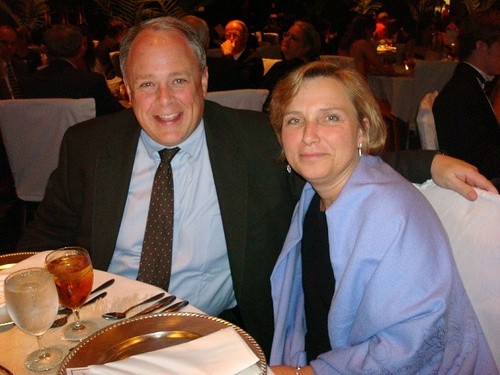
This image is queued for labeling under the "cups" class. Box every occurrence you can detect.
[379,39,385,48]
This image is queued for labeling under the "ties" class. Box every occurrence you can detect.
[135,147,183,293]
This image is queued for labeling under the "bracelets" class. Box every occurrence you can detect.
[294,365,301,374]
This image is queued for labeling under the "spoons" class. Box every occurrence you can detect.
[49,292,107,328]
[102,293,165,320]
[58,279,115,314]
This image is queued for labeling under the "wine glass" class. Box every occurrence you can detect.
[4,267,66,372]
[45,247,98,342]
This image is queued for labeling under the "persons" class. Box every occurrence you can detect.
[15,17,498,364]
[0,0,500,193]
[269,60,499,374]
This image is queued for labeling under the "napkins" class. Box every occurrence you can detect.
[67,327,260,375]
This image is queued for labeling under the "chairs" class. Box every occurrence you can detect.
[0,60,500,375]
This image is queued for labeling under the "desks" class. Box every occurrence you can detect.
[0,263,206,375]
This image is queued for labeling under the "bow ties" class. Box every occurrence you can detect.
[484,79,496,95]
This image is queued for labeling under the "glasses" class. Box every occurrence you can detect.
[282,30,302,43]
